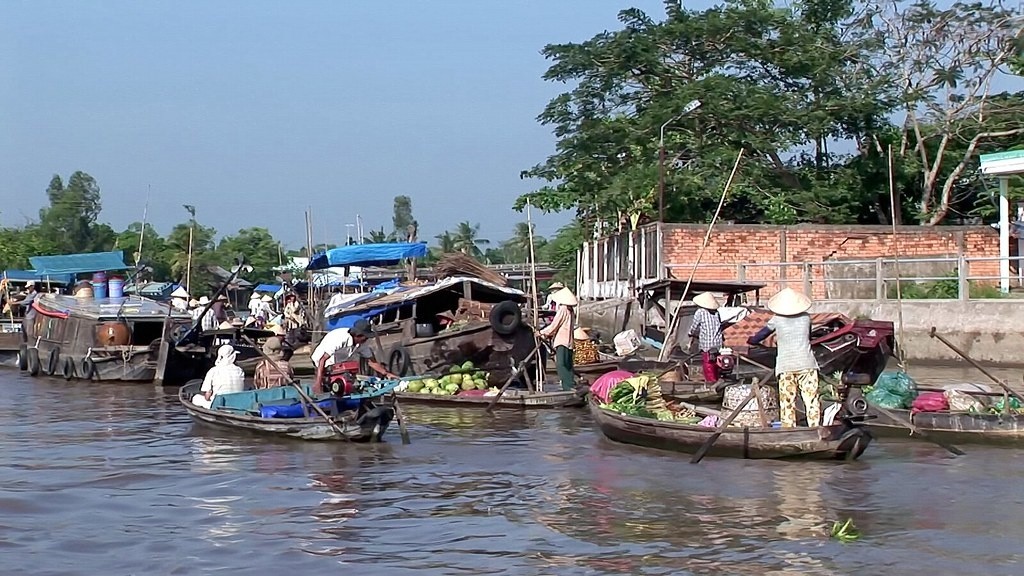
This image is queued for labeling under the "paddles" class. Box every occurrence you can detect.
[239,328,356,448]
[690,368,776,466]
[534,331,581,378]
[486,335,549,412]
[929,326,1024,403]
[368,319,411,444]
[752,340,967,457]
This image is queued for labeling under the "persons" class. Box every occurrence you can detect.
[688,290,722,395]
[261,325,295,362]
[184,293,232,334]
[534,281,579,393]
[747,286,823,429]
[310,320,399,397]
[246,291,298,325]
[192,344,246,412]
[0,281,37,318]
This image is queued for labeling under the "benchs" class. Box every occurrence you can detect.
[211,384,314,410]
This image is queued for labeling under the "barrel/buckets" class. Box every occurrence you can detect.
[92,273,124,298]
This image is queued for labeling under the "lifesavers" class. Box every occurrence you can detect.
[385,346,409,377]
[489,300,520,334]
[19,344,95,380]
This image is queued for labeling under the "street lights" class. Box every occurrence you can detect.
[657,98,703,220]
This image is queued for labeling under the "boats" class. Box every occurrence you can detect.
[307,240,548,386]
[393,386,578,408]
[0,270,78,350]
[578,372,873,461]
[19,250,204,382]
[178,374,399,443]
[857,386,1024,444]
[673,303,896,388]
[205,325,314,370]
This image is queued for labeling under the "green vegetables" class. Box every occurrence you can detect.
[599,374,674,421]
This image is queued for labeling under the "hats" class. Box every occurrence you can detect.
[217,295,227,300]
[261,294,273,301]
[214,345,241,367]
[250,292,260,298]
[197,295,211,305]
[25,280,35,287]
[285,290,299,298]
[352,319,375,338]
[243,316,255,327]
[232,317,244,326]
[692,292,720,310]
[436,310,456,322]
[767,286,812,316]
[551,287,579,306]
[171,284,190,298]
[263,336,284,361]
[573,327,589,340]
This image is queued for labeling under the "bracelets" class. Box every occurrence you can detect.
[383,368,390,376]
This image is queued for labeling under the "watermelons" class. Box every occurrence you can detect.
[407,362,489,394]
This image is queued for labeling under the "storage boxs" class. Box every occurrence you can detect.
[612,329,642,355]
